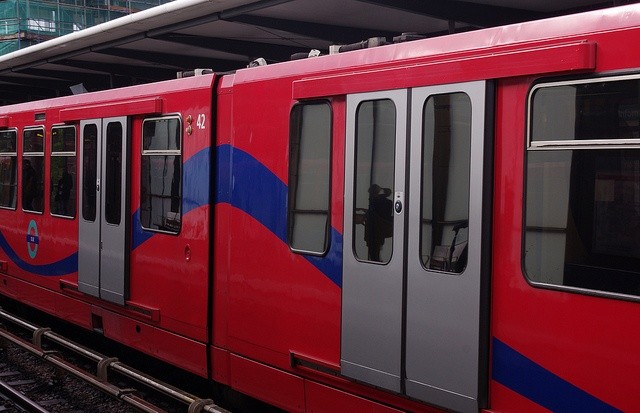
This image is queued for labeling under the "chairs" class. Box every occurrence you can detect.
[429,235,468,273]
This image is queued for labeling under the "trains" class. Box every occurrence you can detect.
[0,3,640,413]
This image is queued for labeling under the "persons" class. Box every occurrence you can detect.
[367,183,392,262]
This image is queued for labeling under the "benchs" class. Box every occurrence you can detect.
[153,214,180,232]
